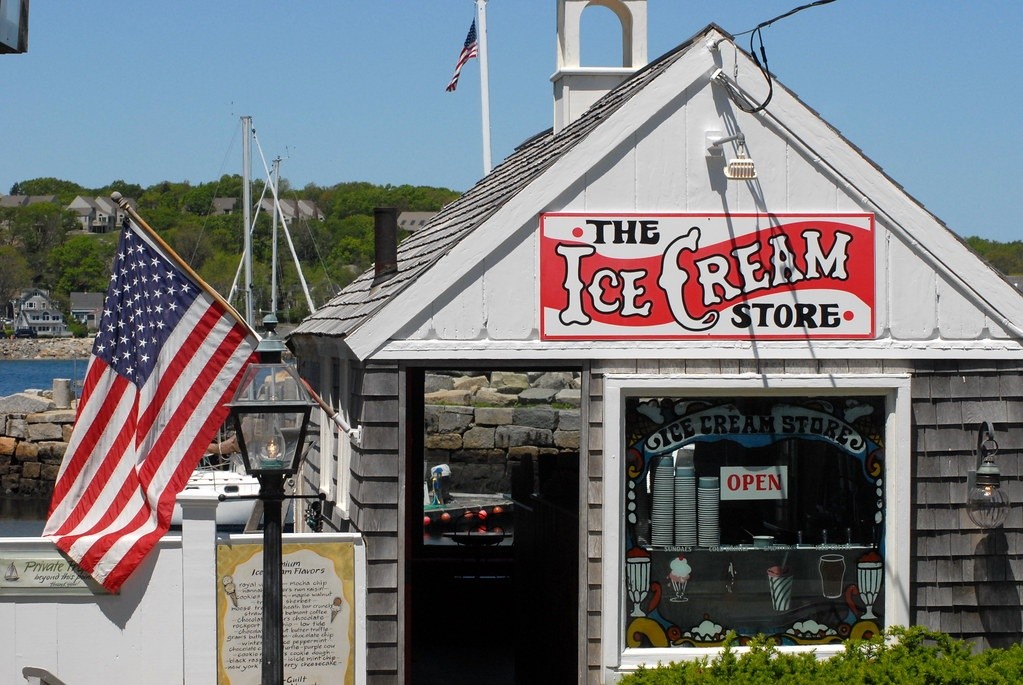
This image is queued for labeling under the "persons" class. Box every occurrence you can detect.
[512,510,576,685]
[197,384,252,471]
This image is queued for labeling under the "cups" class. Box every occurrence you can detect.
[768,575,794,611]
[819,555,846,598]
[858,562,882,619]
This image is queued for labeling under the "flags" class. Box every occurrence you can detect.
[42,217,262,595]
[445,17,478,92]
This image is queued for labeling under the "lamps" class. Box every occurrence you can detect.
[968,420,1010,530]
[219,312,329,502]
[705,129,758,180]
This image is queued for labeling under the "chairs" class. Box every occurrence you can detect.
[452,512,505,585]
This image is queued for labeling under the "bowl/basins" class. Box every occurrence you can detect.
[698,477,720,547]
[753,536,774,548]
[674,467,696,547]
[651,456,674,547]
[633,477,649,546]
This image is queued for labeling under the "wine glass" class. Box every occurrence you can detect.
[670,579,688,602]
[627,558,651,617]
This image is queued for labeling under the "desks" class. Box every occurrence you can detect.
[442,532,513,586]
